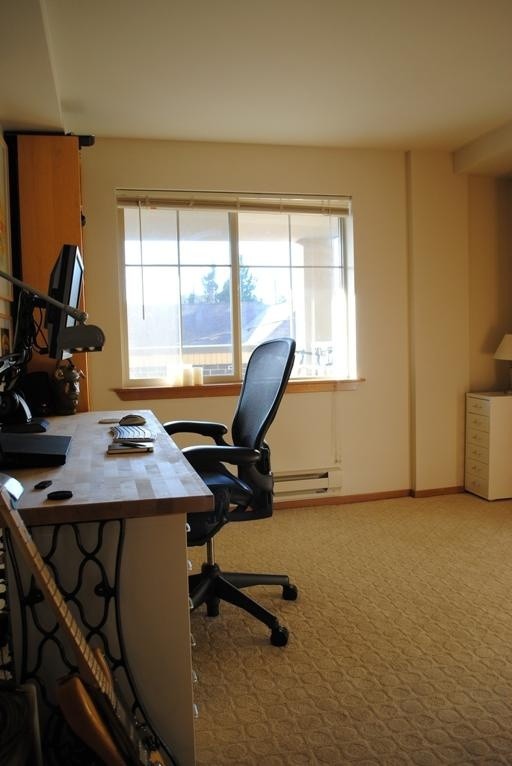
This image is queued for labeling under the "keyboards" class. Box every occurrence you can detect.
[110,426,157,442]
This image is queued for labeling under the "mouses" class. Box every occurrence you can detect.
[119,414,146,426]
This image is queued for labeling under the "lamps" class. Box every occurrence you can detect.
[492,334,512,362]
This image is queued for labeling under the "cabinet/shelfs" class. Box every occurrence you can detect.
[16,135,90,413]
[463,392,512,501]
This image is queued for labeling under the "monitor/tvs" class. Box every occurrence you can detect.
[46,244,84,361]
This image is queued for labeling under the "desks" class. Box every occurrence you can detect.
[0,408,215,766]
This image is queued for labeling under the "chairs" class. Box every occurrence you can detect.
[163,337,297,646]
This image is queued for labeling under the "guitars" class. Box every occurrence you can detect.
[0,485,168,765]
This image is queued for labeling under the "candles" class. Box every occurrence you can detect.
[172,364,203,386]
